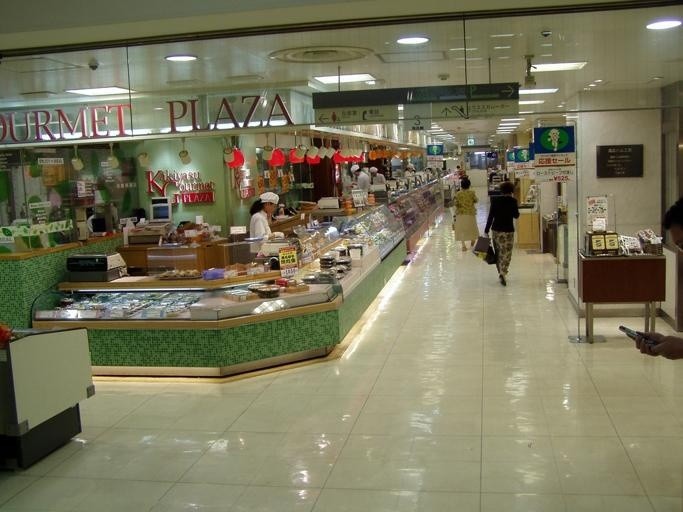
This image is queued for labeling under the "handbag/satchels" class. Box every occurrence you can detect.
[473,237,496,264]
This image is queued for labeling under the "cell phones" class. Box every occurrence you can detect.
[619,325,658,346]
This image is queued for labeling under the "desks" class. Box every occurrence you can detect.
[577,248,665,344]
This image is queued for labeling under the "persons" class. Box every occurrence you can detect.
[453,165,465,191]
[250,191,280,238]
[626,198,683,360]
[346,164,369,191]
[486,160,501,177]
[404,164,416,177]
[485,182,519,286]
[87,206,96,232]
[370,167,386,185]
[453,179,478,251]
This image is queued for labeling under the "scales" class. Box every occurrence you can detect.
[66,252,127,281]
[256,239,300,256]
[317,197,339,209]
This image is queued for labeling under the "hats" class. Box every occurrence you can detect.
[351,165,360,174]
[407,164,415,171]
[259,191,279,204]
[370,167,378,173]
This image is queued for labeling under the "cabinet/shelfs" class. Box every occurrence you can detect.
[31,173,446,383]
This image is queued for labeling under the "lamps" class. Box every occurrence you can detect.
[71,131,361,172]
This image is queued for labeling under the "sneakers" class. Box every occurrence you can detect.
[499,274,506,285]
[462,240,475,251]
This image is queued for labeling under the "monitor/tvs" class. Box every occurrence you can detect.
[149,203,172,223]
